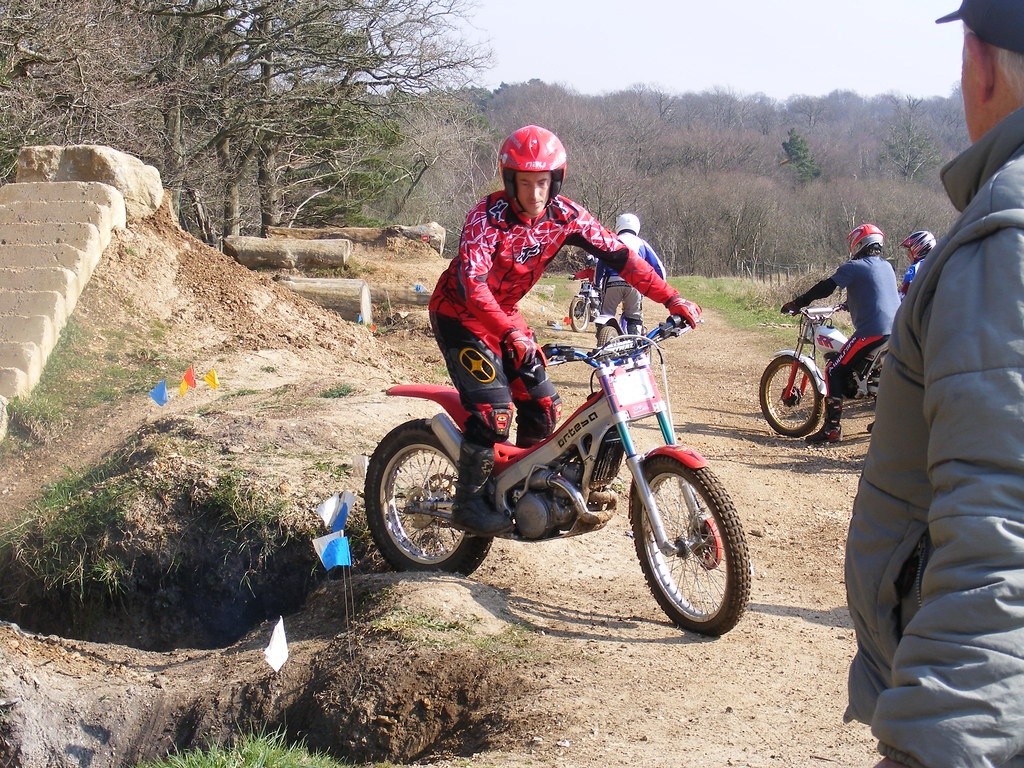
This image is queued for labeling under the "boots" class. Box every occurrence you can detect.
[447,437,515,537]
[515,432,547,449]
[803,393,843,441]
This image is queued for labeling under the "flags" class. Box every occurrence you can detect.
[152,368,220,407]
[264,616,289,671]
[314,489,358,572]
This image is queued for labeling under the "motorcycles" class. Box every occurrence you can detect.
[360,306,752,639]
[567,274,603,334]
[592,299,654,364]
[759,303,896,442]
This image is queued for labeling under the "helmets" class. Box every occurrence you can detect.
[497,121,567,201]
[584,255,598,269]
[900,231,937,262]
[615,214,642,236]
[846,222,884,258]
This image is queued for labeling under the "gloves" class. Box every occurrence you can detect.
[568,274,575,280]
[502,329,548,369]
[667,298,702,330]
[781,296,804,318]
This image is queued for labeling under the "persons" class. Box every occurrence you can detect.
[568,255,595,283]
[898,231,937,301]
[428,125,702,537]
[843,0,1024,768]
[593,213,667,335]
[781,224,902,443]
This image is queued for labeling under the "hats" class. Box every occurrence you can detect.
[936,0,1024,56]
